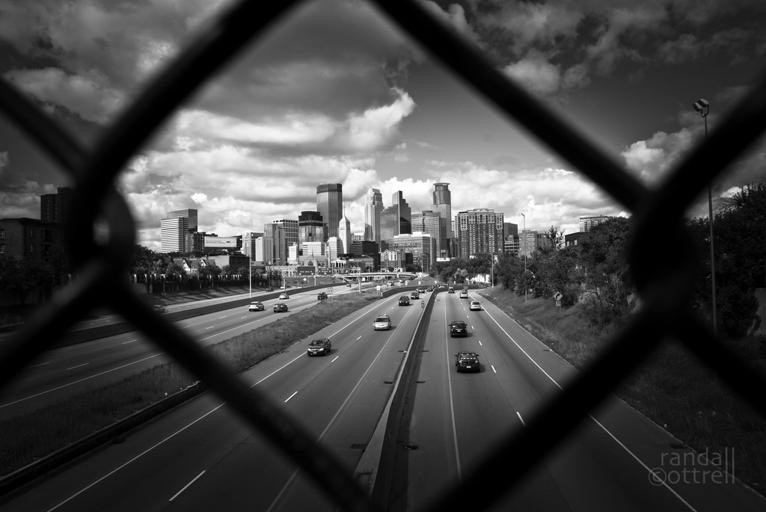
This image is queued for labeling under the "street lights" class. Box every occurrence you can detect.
[694,97,720,327]
[520,212,526,296]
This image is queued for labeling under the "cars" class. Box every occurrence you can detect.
[460,290,468,298]
[448,286,454,293]
[470,300,481,310]
[455,352,481,372]
[450,321,468,336]
[373,314,391,330]
[274,303,288,312]
[416,287,425,293]
[398,295,409,306]
[249,300,264,310]
[307,338,331,357]
[279,293,289,299]
[318,292,327,300]
[411,292,419,299]
